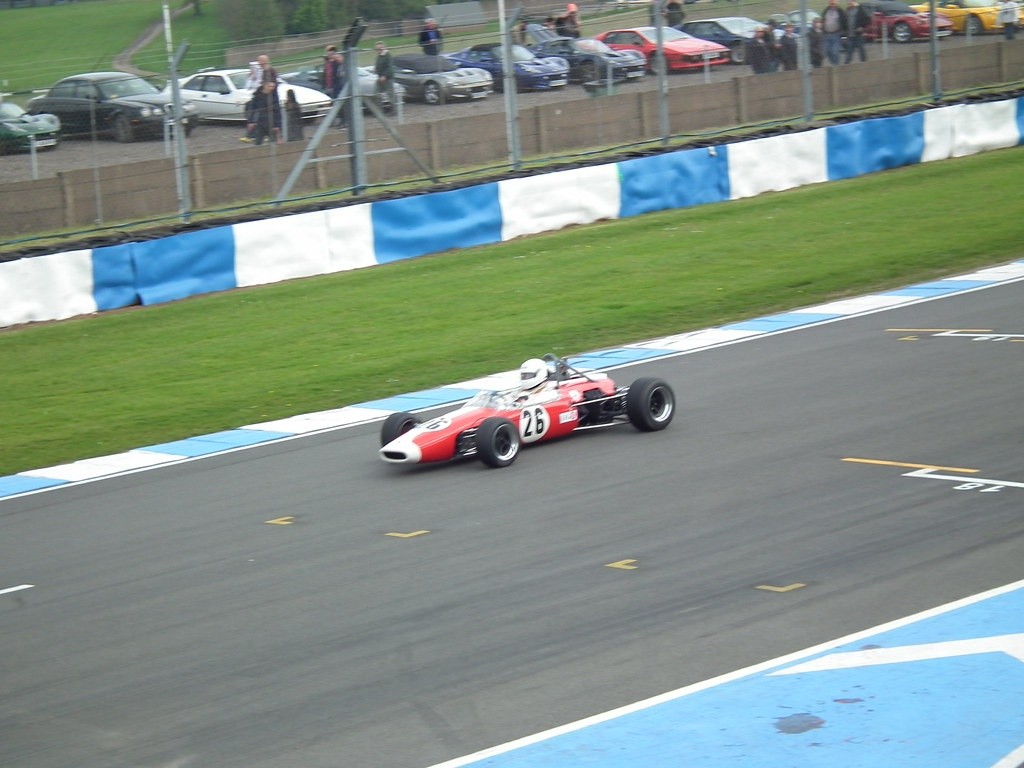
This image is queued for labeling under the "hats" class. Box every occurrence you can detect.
[565,3,578,13]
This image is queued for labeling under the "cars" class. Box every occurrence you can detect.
[18,69,196,143]
[1,100,64,154]
[155,68,333,130]
[345,51,495,106]
[277,63,402,115]
[587,26,732,74]
[430,39,569,93]
[524,39,649,83]
[909,0,1024,35]
[824,0,957,47]
[666,18,802,64]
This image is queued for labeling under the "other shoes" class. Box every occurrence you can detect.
[239,136,270,143]
[386,110,397,117]
[335,121,349,129]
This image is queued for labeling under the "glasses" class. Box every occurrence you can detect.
[756,31,763,33]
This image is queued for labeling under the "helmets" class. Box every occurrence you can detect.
[519,357,548,392]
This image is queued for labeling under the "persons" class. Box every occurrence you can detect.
[417,17,444,56]
[374,40,396,117]
[323,45,352,129]
[995,0,1019,40]
[511,358,548,402]
[747,0,869,75]
[240,55,304,144]
[510,4,582,47]
[649,0,685,26]
